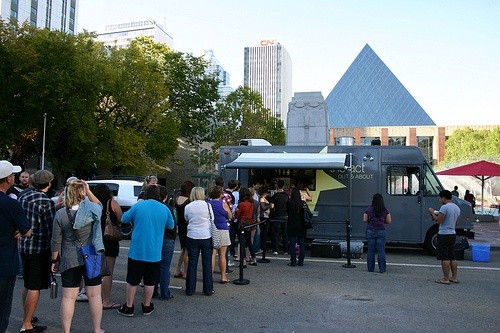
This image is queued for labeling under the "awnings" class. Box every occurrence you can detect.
[226,153,346,170]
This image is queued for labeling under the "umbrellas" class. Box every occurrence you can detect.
[435,160,500,209]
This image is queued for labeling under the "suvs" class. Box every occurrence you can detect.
[49,181,143,240]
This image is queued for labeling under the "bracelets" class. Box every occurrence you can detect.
[51,259,57,263]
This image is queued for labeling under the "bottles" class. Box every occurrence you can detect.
[50,278,58,299]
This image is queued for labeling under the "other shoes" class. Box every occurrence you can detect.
[152,292,159,298]
[76,292,88,302]
[117,303,134,317]
[206,290,215,296]
[31,316,37,323]
[20,325,47,333]
[219,249,263,284]
[186,290,192,295]
[282,251,288,255]
[272,252,278,255]
[159,295,173,300]
[288,263,303,266]
[141,302,155,316]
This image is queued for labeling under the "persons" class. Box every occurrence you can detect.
[215,176,242,273]
[270,180,290,254]
[233,188,260,262]
[118,176,177,316]
[208,186,232,284]
[286,184,312,267]
[184,187,215,296]
[465,190,475,214]
[248,179,274,256]
[428,190,461,285]
[0,160,77,333]
[51,177,103,333]
[92,184,123,309]
[174,181,195,280]
[451,186,459,197]
[234,187,257,269]
[363,193,391,273]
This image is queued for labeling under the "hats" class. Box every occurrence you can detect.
[0,160,21,179]
[32,170,54,183]
[66,177,80,187]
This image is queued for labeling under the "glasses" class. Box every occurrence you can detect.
[11,173,17,176]
[147,175,152,183]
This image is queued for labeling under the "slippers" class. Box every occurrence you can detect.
[103,303,121,310]
[434,278,460,284]
[174,271,182,278]
[183,273,187,279]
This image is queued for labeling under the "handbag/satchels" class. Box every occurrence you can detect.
[80,244,111,279]
[207,201,222,249]
[303,201,313,223]
[168,197,178,225]
[104,199,123,242]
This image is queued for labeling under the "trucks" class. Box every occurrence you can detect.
[216,143,475,258]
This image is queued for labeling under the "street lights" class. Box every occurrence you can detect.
[41,112,47,169]
